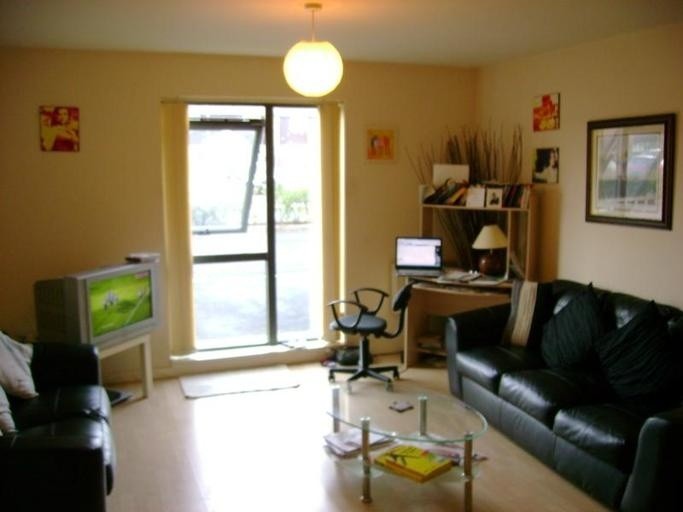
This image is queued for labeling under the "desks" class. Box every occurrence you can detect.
[97,334,155,399]
[396,185,537,372]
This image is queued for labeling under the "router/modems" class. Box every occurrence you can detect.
[123,253,160,263]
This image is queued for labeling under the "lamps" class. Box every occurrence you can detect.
[471,224,508,275]
[277,2,344,99]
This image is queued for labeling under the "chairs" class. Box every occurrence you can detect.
[323,281,411,392]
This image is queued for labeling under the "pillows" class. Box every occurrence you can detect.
[592,300,679,402]
[1,387,20,434]
[537,283,603,367]
[1,332,38,398]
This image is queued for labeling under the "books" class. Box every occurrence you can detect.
[424,177,536,209]
[373,445,453,484]
[325,426,391,458]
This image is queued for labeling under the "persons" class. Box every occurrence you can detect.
[41,107,79,151]
[535,149,557,182]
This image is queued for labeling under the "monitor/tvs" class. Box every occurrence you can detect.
[394,236,442,269]
[32,262,159,353]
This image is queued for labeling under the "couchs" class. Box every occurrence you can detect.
[1,330,116,510]
[442,278,680,509]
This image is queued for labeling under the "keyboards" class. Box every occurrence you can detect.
[396,269,440,277]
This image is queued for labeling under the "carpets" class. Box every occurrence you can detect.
[176,363,299,400]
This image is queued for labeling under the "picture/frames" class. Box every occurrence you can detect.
[363,127,398,164]
[485,187,502,208]
[584,112,675,232]
